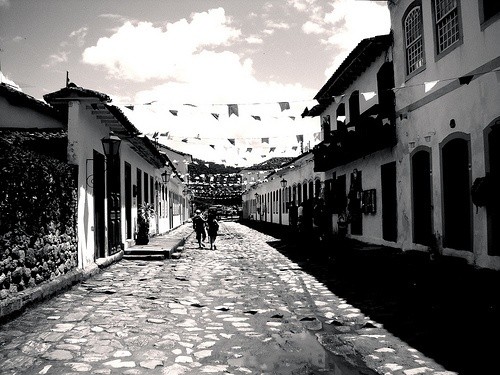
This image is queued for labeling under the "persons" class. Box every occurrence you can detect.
[207,213,220,251]
[192,209,210,250]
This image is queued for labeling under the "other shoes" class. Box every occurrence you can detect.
[198,241,216,250]
